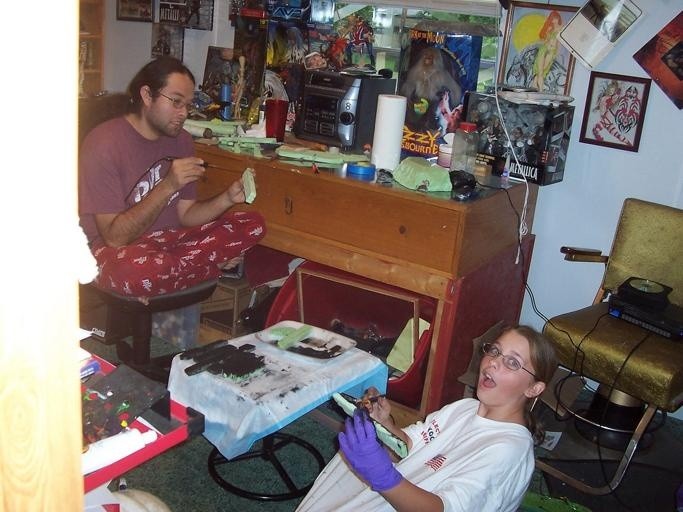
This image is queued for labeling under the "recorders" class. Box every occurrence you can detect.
[294,70,396,155]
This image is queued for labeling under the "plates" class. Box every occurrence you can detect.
[254,320,358,360]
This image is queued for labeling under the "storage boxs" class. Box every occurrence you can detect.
[457,319,586,418]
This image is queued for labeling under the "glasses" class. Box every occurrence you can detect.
[159,91,197,113]
[480,343,540,380]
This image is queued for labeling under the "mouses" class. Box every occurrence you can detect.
[451,170,476,189]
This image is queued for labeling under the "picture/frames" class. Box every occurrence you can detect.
[497,1,581,96]
[579,70,652,152]
[116,0,155,22]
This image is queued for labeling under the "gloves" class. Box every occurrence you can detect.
[335,407,408,494]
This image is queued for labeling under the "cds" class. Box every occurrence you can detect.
[630,279,664,293]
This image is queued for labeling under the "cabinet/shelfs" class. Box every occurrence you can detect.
[79,0,104,99]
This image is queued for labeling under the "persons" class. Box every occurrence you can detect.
[532,12,565,92]
[79,56,267,306]
[294,326,557,512]
[592,81,631,146]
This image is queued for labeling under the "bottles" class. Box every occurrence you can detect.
[449,119,479,171]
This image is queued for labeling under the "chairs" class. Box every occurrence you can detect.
[79,116,215,380]
[528,198,682,497]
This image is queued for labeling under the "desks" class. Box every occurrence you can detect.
[192,142,538,427]
[166,320,389,502]
[79,346,205,512]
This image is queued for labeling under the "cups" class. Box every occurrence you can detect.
[263,98,287,143]
